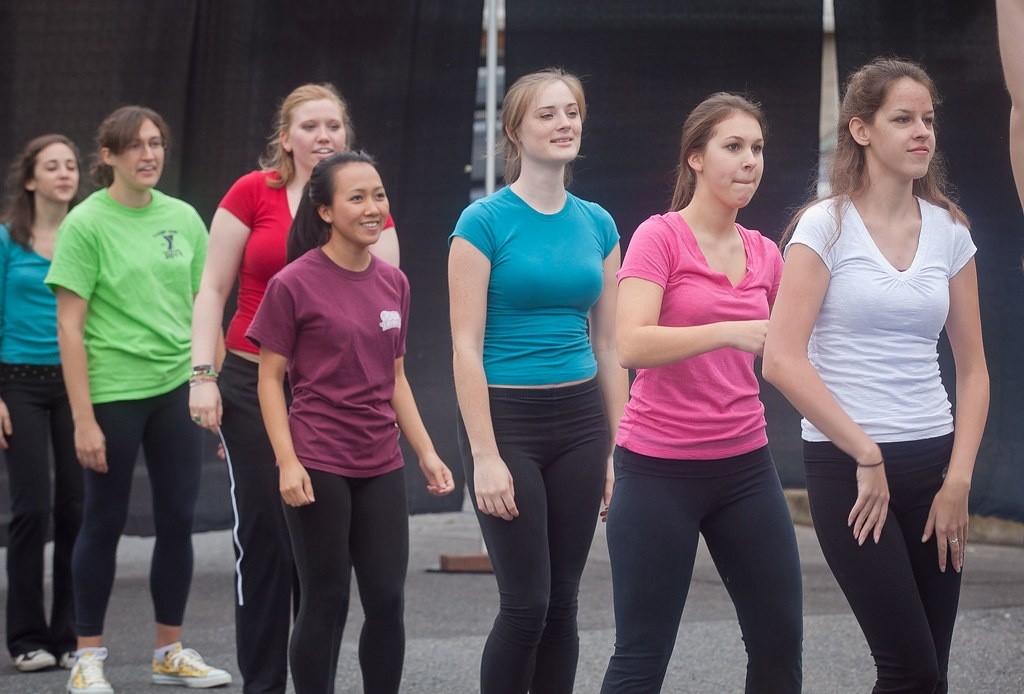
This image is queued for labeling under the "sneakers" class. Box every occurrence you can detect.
[59,652,78,668]
[151,646,232,688]
[66,652,115,694]
[13,649,57,672]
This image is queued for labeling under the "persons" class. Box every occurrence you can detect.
[601,91,803,694]
[187,83,398,694]
[444,70,630,694]
[0,134,85,670]
[763,57,991,694]
[44,106,233,694]
[245,149,453,694]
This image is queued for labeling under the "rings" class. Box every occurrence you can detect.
[192,417,200,422]
[949,538,958,544]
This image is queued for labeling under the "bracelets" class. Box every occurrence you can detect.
[189,365,219,387]
[858,459,883,467]
